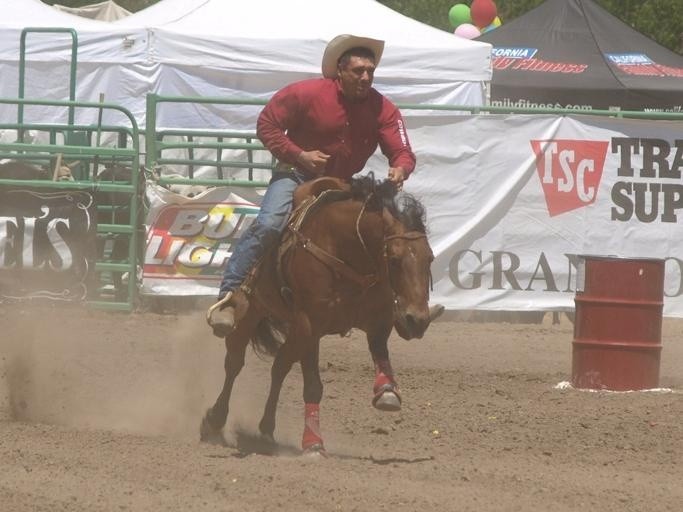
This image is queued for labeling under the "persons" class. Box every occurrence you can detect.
[207,36,446,339]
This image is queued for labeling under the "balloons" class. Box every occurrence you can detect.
[454,23,481,41]
[447,2,473,27]
[478,15,502,35]
[470,0,496,31]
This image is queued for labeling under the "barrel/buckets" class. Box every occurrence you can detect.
[570,255,665,391]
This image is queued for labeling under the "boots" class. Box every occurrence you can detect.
[210,305,238,338]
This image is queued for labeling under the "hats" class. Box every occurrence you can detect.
[322,33,385,78]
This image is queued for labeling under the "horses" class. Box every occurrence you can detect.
[198,170,435,466]
[2,160,195,311]
